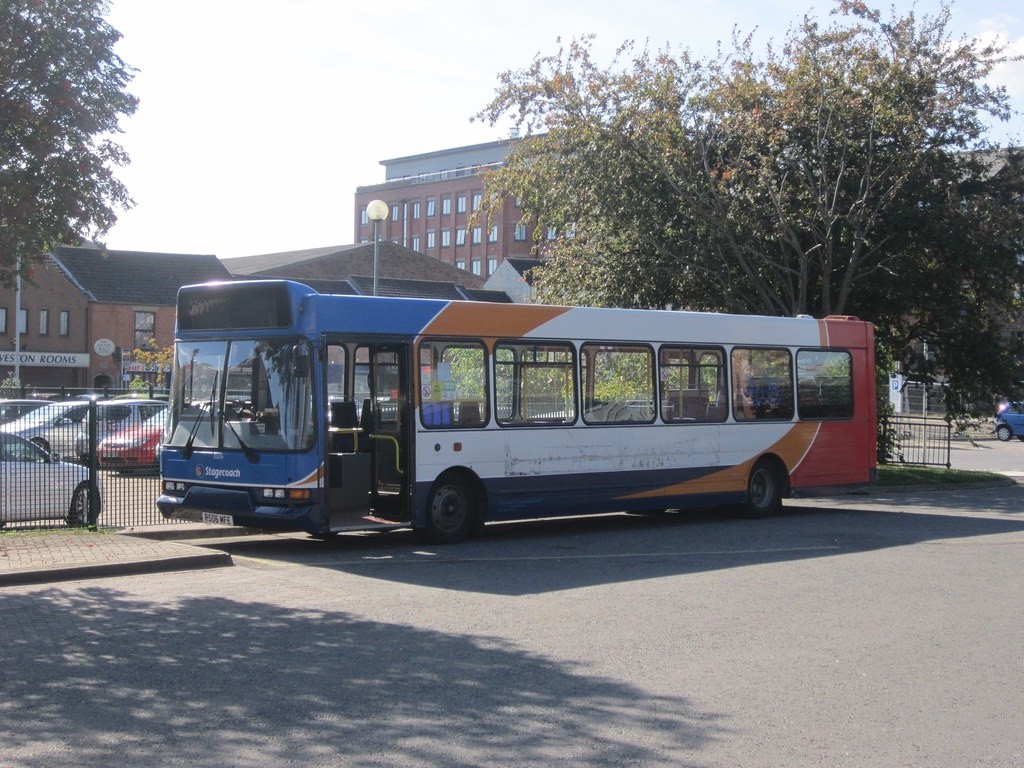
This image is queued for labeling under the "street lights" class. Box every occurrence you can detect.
[365,198,390,390]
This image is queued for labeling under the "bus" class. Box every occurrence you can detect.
[158,274,879,549]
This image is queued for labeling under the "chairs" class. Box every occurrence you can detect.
[459,402,480,426]
[331,395,359,446]
[735,377,851,418]
[348,399,382,444]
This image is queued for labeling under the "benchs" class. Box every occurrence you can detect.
[668,389,710,422]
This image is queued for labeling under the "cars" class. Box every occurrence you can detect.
[0,430,104,530]
[0,391,398,476]
[991,401,1024,443]
